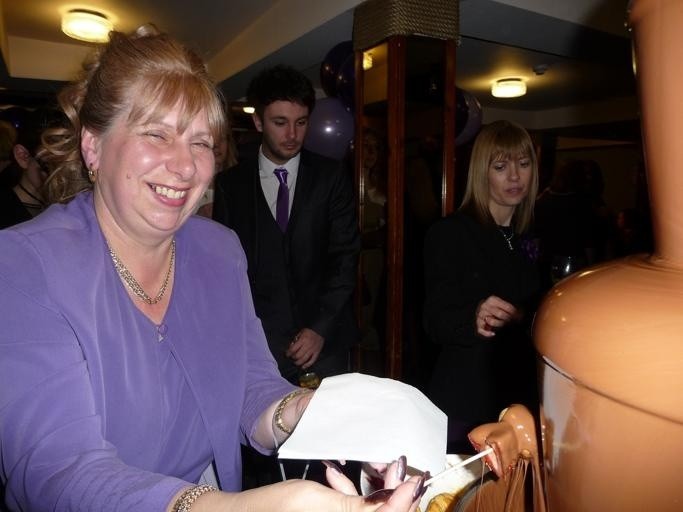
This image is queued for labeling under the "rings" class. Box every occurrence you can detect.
[484,315,493,322]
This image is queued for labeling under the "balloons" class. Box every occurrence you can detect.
[456,94,483,145]
[303,40,355,160]
[456,87,468,137]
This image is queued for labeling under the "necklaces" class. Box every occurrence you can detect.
[502,232,515,251]
[106,239,175,304]
[19,183,47,205]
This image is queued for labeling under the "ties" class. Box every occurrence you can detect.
[273,169,289,233]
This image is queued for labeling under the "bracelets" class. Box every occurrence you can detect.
[275,388,313,433]
[172,484,220,512]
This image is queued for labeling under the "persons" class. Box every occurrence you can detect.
[422,119,555,441]
[0,109,68,227]
[212,63,360,486]
[0,21,429,512]
[201,129,238,219]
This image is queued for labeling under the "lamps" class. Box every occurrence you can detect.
[490,78,528,99]
[60,9,114,44]
